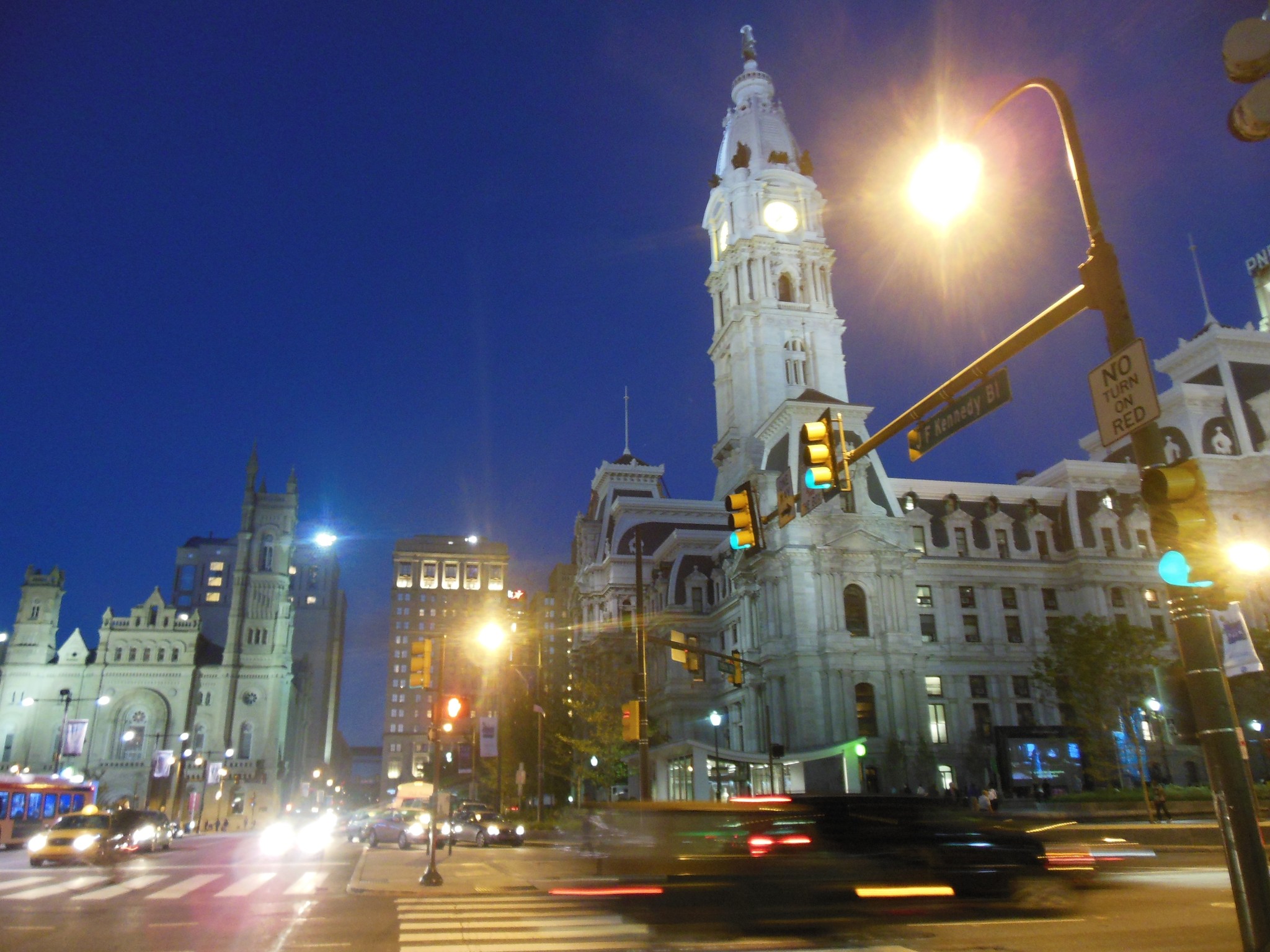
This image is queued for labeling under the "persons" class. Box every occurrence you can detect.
[203,816,248,832]
[1150,780,1172,825]
[899,767,1091,815]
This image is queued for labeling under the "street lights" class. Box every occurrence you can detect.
[476,620,545,823]
[901,74,1270,952]
[1148,697,1173,785]
[122,730,189,810]
[183,748,235,833]
[1251,719,1268,782]
[709,710,722,803]
[590,755,601,802]
[22,688,110,773]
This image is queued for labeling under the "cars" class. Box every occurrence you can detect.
[28,804,184,867]
[346,806,525,849]
[544,801,1100,935]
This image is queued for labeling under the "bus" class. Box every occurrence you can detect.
[0,772,95,848]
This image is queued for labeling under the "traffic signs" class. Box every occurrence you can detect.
[776,466,797,529]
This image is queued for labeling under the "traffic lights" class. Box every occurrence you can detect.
[1221,16,1270,143]
[622,701,640,742]
[800,406,841,503]
[724,480,761,559]
[681,637,698,671]
[442,695,470,721]
[1140,459,1220,587]
[725,650,742,688]
[410,638,432,688]
